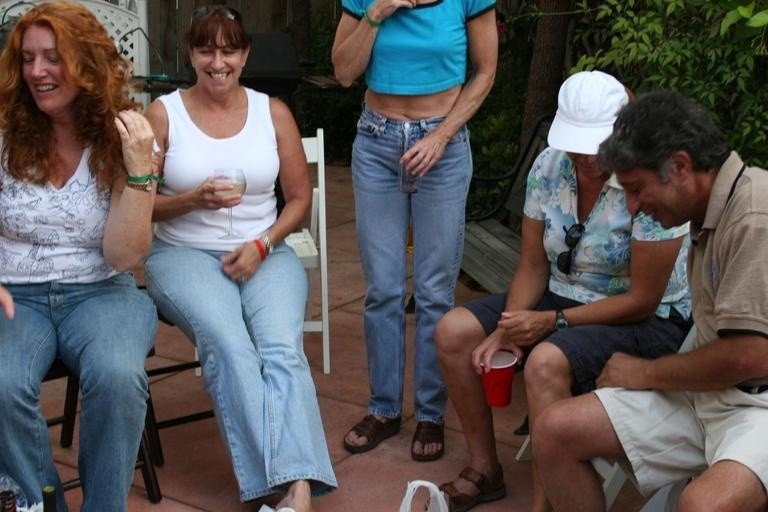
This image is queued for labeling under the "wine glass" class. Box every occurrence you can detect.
[213,166,249,241]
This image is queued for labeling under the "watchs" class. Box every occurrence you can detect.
[263,235,274,255]
[126,180,154,193]
[552,308,569,333]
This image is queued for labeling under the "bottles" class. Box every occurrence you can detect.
[39,487,59,512]
[0,490,18,512]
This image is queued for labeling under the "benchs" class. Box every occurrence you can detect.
[404,114,554,316]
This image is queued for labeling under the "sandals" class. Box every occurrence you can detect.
[343,414,402,453]
[411,419,445,461]
[424,464,507,511]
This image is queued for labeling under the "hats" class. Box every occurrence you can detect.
[547,70,629,157]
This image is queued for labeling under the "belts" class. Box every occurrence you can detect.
[736,383,768,395]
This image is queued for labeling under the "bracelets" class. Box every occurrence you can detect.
[363,10,383,30]
[255,238,267,262]
[128,171,167,185]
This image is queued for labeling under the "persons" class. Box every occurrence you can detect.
[424,68,694,512]
[326,0,502,459]
[0,2,158,512]
[522,88,766,511]
[141,5,339,512]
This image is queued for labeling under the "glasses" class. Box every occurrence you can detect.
[556,224,586,276]
[189,6,243,32]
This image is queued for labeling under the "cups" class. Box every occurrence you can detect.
[476,349,522,408]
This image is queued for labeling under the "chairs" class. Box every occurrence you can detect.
[514,323,704,511]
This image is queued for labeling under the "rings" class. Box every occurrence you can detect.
[242,276,246,283]
